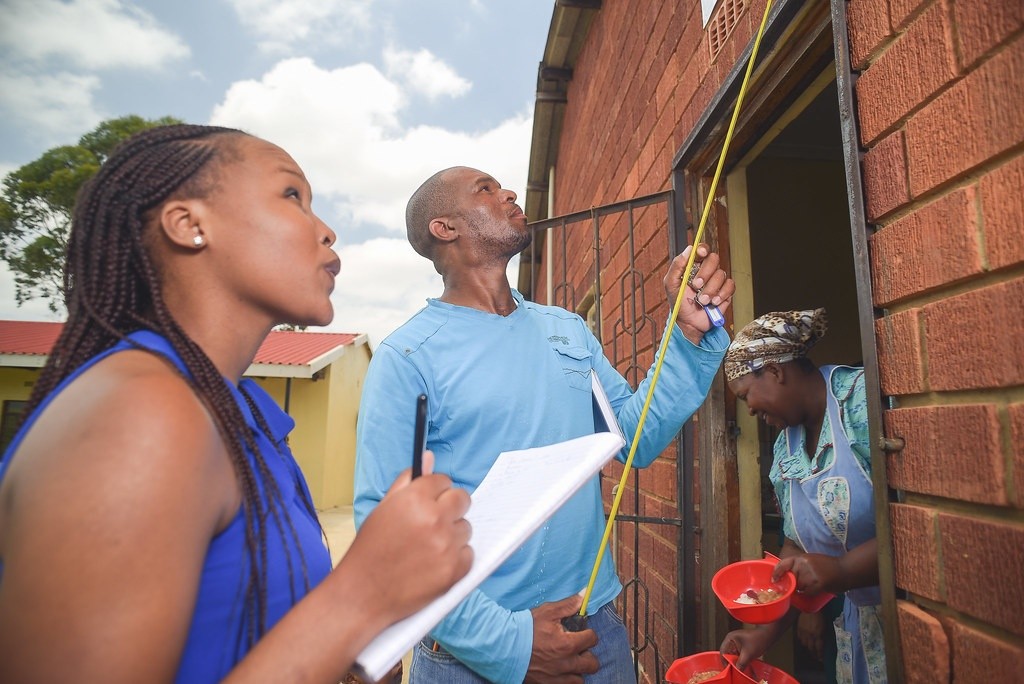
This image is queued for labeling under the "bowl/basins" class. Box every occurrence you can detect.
[711,560,796,624]
[764,550,838,613]
[723,654,800,684]
[665,651,731,684]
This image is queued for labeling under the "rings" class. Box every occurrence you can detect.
[724,270,727,279]
[796,589,803,593]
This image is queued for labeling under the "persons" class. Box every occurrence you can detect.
[719,310,890,684]
[353,167,737,684]
[0,123,474,684]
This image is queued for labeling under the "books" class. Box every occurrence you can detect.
[587,367,627,446]
[354,432,624,684]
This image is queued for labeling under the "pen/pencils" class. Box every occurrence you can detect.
[411,393,428,482]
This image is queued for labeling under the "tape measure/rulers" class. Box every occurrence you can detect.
[561,0,771,631]
